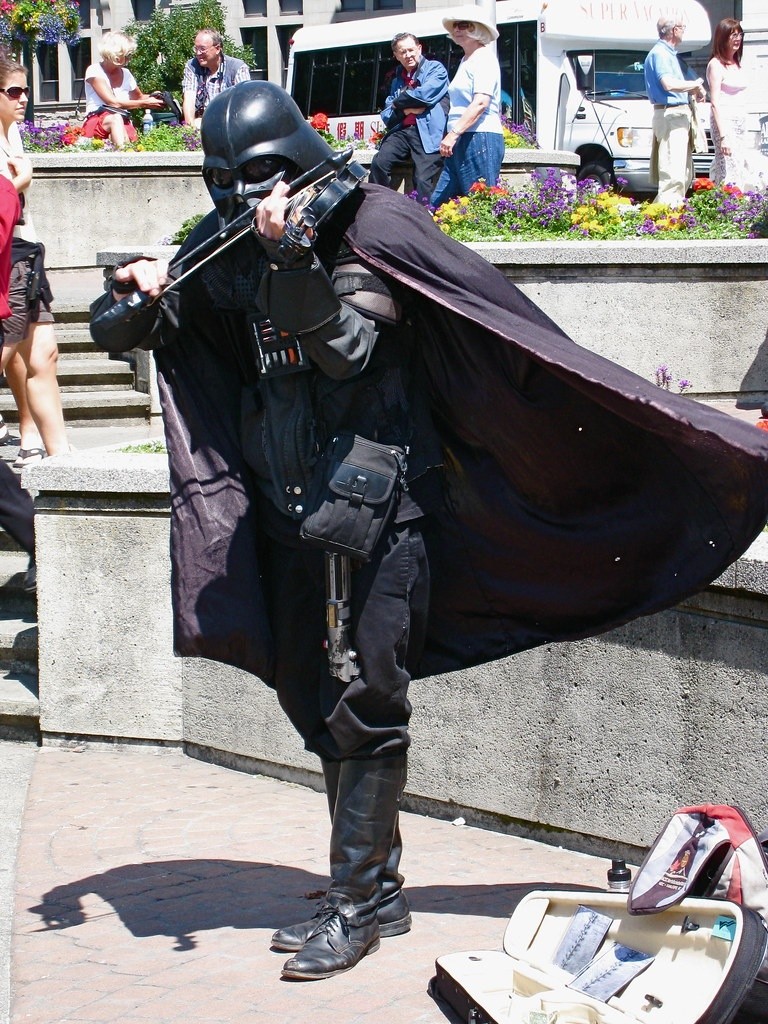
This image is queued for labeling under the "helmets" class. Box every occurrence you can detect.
[198,80,343,219]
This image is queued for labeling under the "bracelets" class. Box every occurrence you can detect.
[452,130,458,134]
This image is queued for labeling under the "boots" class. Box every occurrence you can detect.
[283,752,408,978]
[271,759,410,951]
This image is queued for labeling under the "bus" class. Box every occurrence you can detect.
[285,0,714,194]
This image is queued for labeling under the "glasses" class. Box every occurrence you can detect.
[452,20,470,31]
[193,42,221,55]
[674,24,686,31]
[0,87,31,99]
[730,32,744,41]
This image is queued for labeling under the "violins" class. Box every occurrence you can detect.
[277,158,372,252]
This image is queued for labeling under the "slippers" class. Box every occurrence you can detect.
[13,446,47,467]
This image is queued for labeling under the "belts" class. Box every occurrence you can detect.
[653,102,685,109]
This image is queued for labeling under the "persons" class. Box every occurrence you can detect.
[705,20,747,191]
[0,459,37,591]
[644,14,706,204]
[428,5,505,208]
[0,48,79,468]
[80,31,166,145]
[181,29,251,128]
[367,32,451,203]
[87,82,767,980]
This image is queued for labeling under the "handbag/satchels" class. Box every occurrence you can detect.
[94,104,131,123]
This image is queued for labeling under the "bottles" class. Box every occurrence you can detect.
[143,109,153,135]
[605,857,633,892]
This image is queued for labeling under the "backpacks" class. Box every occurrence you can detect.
[625,803,768,986]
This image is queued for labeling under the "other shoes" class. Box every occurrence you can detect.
[0,420,9,446]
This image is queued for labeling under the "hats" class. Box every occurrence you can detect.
[442,5,499,41]
